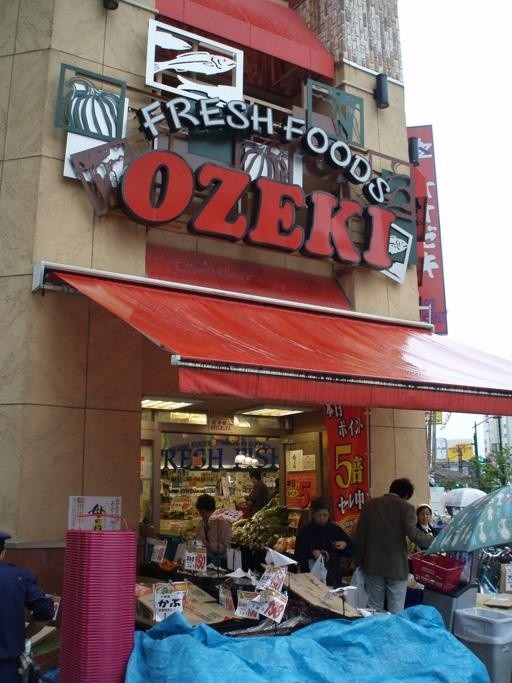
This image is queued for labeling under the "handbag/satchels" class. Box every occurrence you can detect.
[308,554,327,585]
[350,567,369,608]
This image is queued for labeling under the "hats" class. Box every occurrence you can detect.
[0,531,12,545]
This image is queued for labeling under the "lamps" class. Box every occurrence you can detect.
[407,134,420,168]
[372,71,389,108]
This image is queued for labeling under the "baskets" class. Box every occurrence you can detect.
[408,550,466,592]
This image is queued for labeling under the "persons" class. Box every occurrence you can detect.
[413,501,444,540]
[1,528,61,681]
[355,476,438,614]
[187,493,235,564]
[294,495,356,592]
[244,468,268,516]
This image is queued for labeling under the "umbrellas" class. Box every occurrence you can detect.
[420,481,511,556]
[439,485,488,508]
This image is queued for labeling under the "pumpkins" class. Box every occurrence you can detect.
[63,77,121,139]
